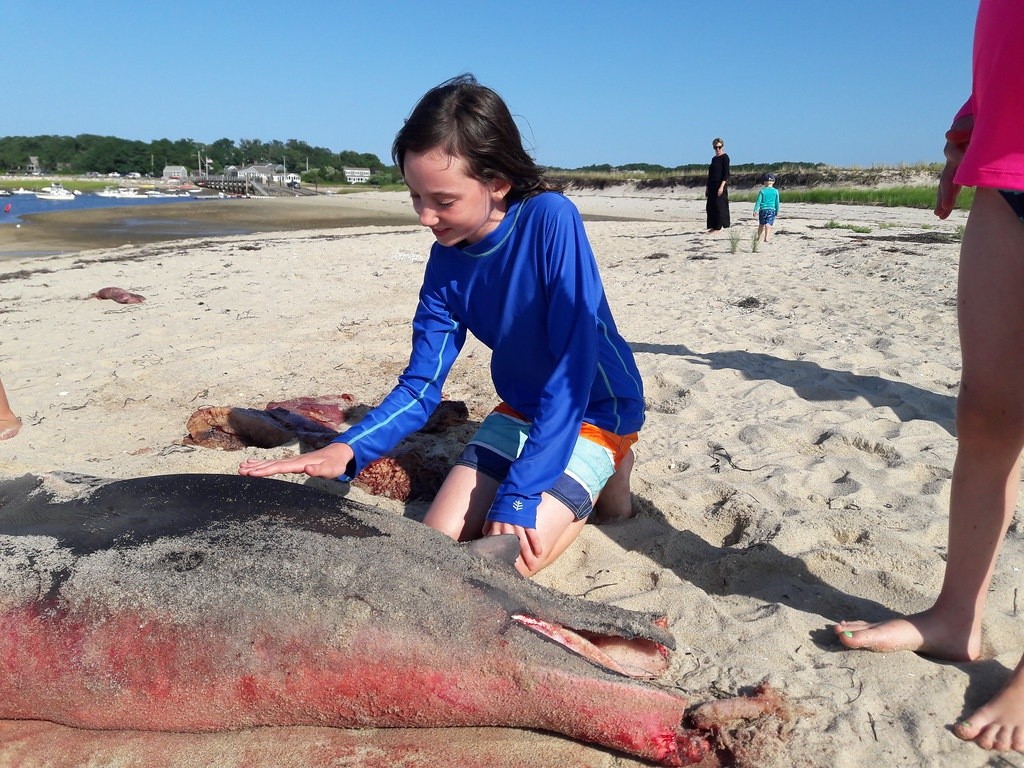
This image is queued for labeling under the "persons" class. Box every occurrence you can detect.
[705,138,730,234]
[753,174,779,242]
[835,0,1024,750]
[238,72,647,579]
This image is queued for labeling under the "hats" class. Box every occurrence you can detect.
[763,173,775,181]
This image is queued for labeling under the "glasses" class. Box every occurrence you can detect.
[714,145,723,149]
